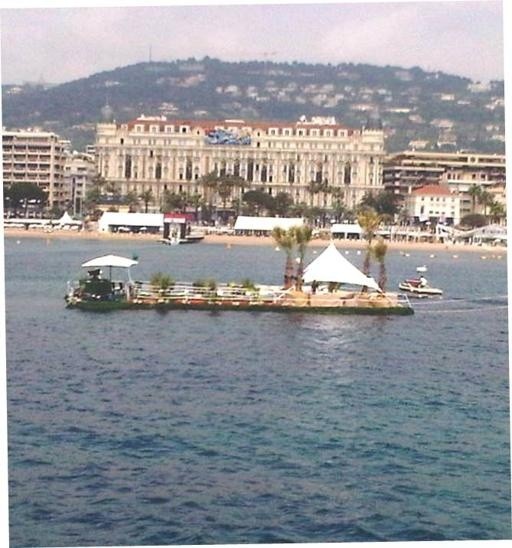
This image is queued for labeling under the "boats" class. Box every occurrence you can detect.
[398,280,444,296]
[157,235,205,245]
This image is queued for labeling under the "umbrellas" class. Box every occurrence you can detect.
[80,254,140,280]
